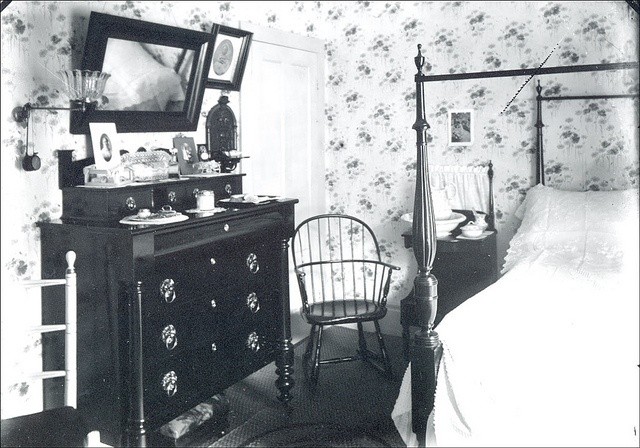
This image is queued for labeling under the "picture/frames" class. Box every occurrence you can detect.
[204,21,255,93]
[88,122,123,171]
[447,108,475,147]
[67,10,215,134]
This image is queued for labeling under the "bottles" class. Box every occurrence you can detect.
[474,213,488,233]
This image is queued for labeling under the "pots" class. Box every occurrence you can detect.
[460,221,483,237]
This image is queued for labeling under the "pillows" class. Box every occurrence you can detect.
[500,181,639,277]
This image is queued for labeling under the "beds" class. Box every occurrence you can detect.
[390,43,639,448]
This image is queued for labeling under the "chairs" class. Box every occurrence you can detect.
[0,249,121,448]
[291,212,401,394]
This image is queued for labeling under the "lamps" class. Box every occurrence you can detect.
[10,67,112,171]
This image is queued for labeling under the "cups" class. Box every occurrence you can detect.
[196,190,215,210]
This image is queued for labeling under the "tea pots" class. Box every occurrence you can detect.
[432,181,458,220]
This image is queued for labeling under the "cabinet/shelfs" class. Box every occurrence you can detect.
[35,148,300,448]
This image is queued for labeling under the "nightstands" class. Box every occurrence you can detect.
[401,159,498,361]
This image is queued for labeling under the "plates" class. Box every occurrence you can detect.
[118,214,190,226]
[456,231,494,241]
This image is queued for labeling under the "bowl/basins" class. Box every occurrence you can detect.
[52,68,112,105]
[400,212,467,237]
